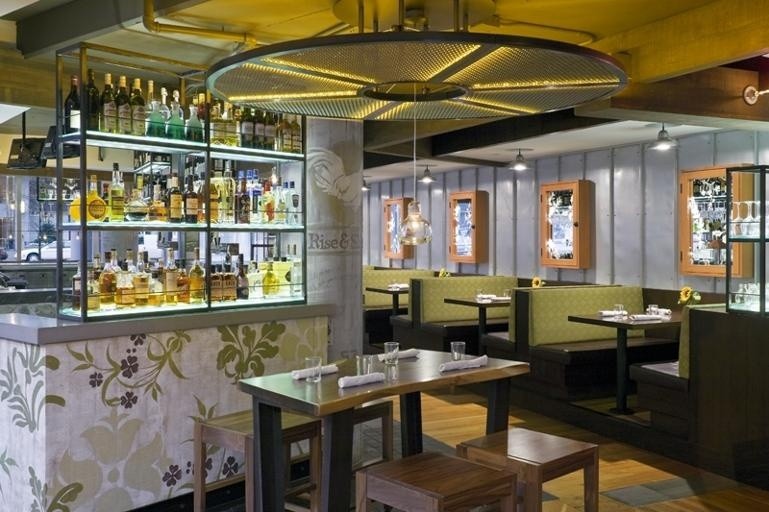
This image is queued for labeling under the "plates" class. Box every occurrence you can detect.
[389,283,410,289]
[478,288,512,301]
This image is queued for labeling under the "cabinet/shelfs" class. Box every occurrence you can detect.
[537,179,593,270]
[726,164,769,316]
[382,197,414,260]
[447,191,489,265]
[56,41,306,323]
[677,163,755,280]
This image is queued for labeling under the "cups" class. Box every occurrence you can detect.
[613,303,626,321]
[355,353,374,379]
[383,341,400,366]
[648,303,658,318]
[450,341,466,362]
[303,354,325,384]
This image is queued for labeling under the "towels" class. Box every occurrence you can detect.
[337,372,384,388]
[291,364,338,380]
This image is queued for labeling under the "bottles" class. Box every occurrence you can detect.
[63,67,304,156]
[69,239,304,312]
[735,276,768,309]
[68,160,303,227]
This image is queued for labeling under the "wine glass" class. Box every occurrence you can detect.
[732,197,769,239]
[692,200,727,224]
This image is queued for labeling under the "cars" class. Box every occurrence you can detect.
[12,239,72,263]
[0,247,8,262]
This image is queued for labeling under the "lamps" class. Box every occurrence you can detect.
[507,147,530,171]
[397,80,432,247]
[648,122,678,151]
[360,180,371,191]
[417,164,436,184]
